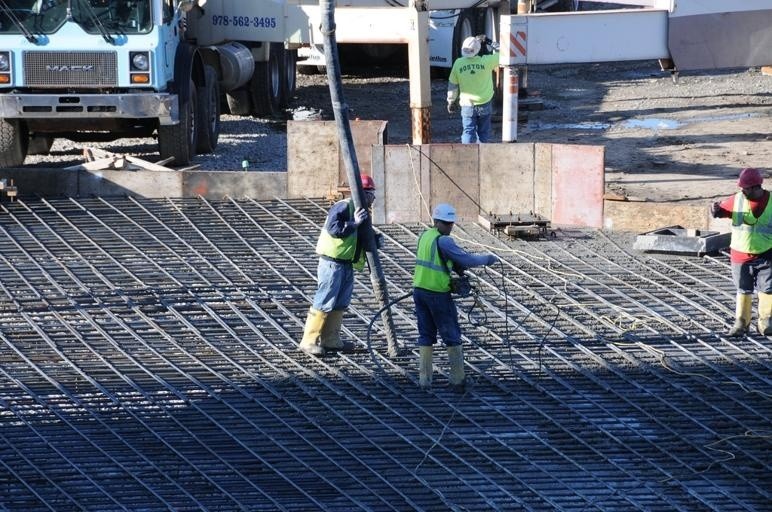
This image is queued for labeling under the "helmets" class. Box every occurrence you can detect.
[360,173,376,190]
[431,204,457,223]
[737,167,763,189]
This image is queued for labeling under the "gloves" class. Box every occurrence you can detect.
[476,34,494,48]
[485,255,499,266]
[446,103,459,115]
[710,201,721,218]
[376,233,384,249]
[354,206,368,227]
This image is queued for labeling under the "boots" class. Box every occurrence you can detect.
[297,306,327,355]
[418,345,434,391]
[757,290,772,337]
[445,344,466,394]
[727,292,754,337]
[318,310,345,350]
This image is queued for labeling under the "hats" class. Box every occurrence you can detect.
[460,37,482,58]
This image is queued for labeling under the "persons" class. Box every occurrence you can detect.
[709,166,772,338]
[411,204,502,395]
[445,32,500,144]
[296,172,384,358]
